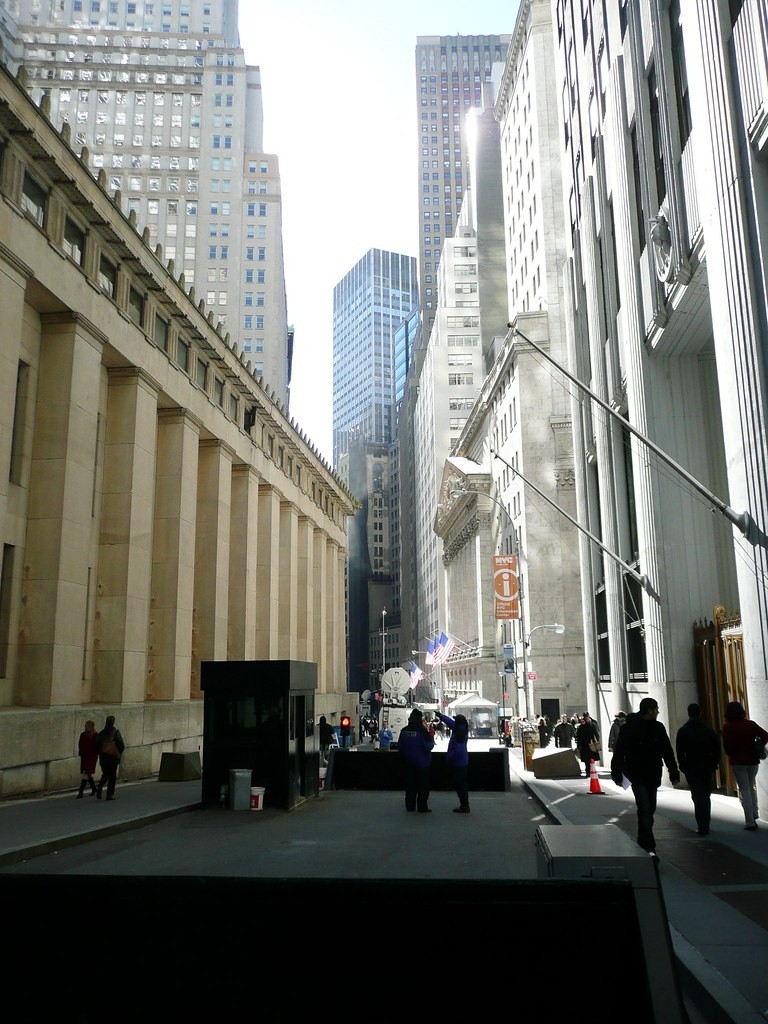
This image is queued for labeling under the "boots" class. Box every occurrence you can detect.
[76,779,87,799]
[88,777,97,796]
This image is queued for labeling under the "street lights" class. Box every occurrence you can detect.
[523,624,565,721]
[449,490,531,721]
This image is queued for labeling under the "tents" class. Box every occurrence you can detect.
[447,693,499,711]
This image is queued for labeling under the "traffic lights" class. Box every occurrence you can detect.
[340,716,350,736]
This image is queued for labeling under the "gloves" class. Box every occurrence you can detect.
[611,771,622,786]
[669,770,679,785]
[434,711,442,717]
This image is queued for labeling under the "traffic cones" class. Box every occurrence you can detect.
[587,758,605,794]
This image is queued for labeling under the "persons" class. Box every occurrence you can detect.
[721,702,768,831]
[576,717,601,778]
[675,703,722,834]
[427,719,450,740]
[373,721,393,751]
[398,709,434,814]
[78,715,125,799]
[359,716,379,743]
[434,711,471,814]
[499,716,513,748]
[316,717,333,766]
[609,698,680,862]
[538,712,600,748]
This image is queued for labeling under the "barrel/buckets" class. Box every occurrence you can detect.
[250,787,266,811]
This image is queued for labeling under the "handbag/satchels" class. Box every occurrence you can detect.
[758,746,767,759]
[589,735,600,752]
[374,741,380,750]
[103,729,121,759]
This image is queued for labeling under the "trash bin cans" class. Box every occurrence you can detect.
[228,769,253,811]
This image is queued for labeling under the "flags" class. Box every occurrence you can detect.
[409,663,427,689]
[426,632,455,666]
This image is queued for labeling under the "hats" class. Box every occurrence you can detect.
[453,714,467,724]
[410,709,422,719]
[615,712,627,718]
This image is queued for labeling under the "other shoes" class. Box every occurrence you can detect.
[745,822,759,830]
[407,806,416,812]
[97,789,102,799]
[418,806,432,812]
[454,805,470,813]
[106,796,115,801]
